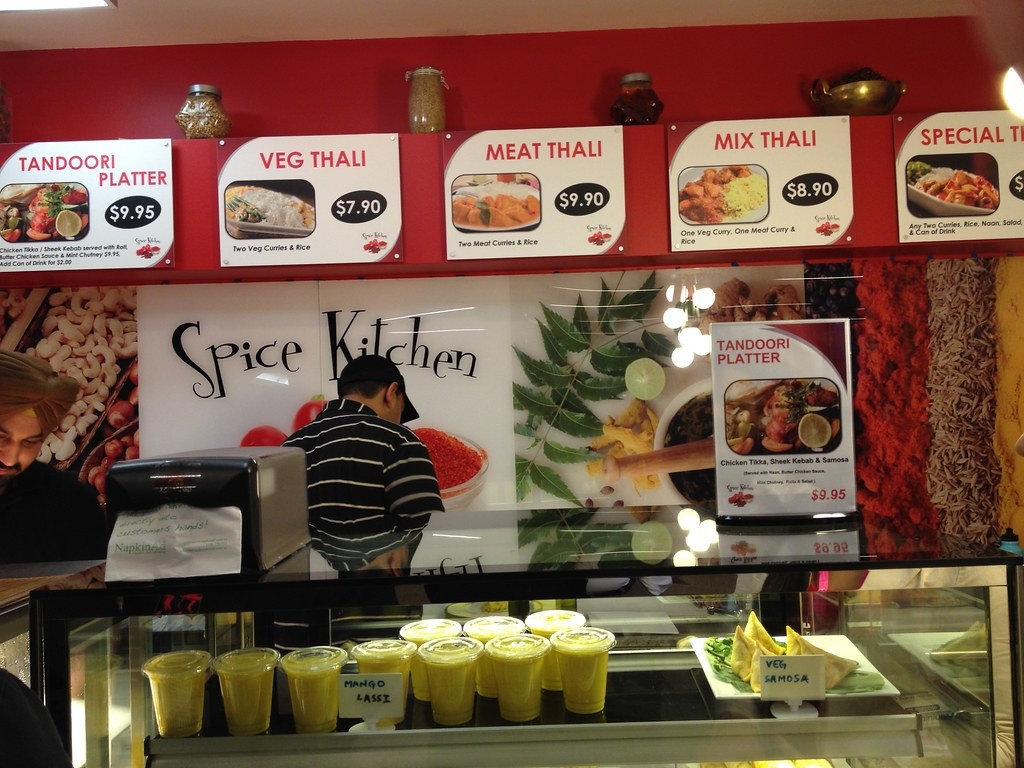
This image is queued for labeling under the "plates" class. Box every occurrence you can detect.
[885,631,988,694]
[907,171,1000,215]
[453,183,542,231]
[688,633,901,700]
[225,193,316,236]
[445,602,543,619]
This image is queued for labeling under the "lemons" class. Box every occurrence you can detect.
[798,413,832,448]
[625,358,666,400]
[56,210,82,237]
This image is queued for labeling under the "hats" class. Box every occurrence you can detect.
[0,349,80,442]
[338,354,419,424]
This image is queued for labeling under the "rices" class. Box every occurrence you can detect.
[241,190,307,228]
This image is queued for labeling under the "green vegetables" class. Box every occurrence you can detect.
[35,185,71,218]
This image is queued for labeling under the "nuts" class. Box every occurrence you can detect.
[0,287,139,507]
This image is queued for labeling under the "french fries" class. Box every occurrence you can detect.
[582,399,662,489]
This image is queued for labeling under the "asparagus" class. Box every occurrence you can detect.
[225,196,266,222]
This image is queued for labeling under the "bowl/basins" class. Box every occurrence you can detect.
[429,429,490,510]
[811,77,907,114]
[654,377,712,505]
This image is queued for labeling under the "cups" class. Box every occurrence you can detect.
[141,608,617,740]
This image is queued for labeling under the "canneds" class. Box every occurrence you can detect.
[405,66,450,134]
[173,83,233,138]
[610,72,663,125]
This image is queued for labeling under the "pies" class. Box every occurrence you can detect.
[730,611,861,694]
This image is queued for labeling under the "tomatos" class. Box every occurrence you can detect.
[240,396,330,448]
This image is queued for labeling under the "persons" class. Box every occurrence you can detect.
[0,350,109,562]
[277,354,446,578]
